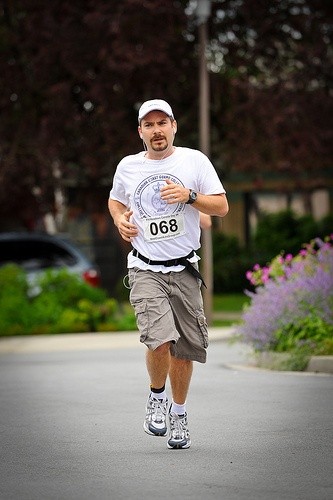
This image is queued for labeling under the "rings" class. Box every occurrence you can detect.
[172,194,176,197]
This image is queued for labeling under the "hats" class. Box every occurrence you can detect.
[138,99,174,125]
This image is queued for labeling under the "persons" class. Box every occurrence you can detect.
[108,100,229,450]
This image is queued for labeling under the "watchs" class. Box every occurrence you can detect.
[185,189,198,205]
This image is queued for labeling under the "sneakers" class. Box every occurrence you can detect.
[165,403,191,450]
[144,393,168,437]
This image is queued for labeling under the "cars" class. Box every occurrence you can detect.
[0,231,103,298]
